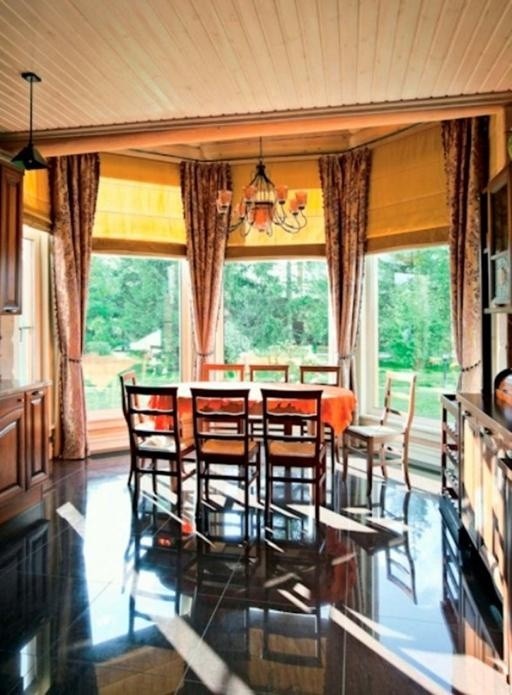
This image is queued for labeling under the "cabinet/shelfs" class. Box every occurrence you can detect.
[0,157,24,316]
[1,386,53,523]
[436,163,512,682]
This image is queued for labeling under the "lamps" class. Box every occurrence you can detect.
[6,70,55,169]
[207,129,309,238]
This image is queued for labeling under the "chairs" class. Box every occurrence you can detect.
[124,492,417,670]
[118,363,357,485]
[342,372,417,490]
[190,387,261,508]
[124,385,196,506]
[260,388,326,509]
